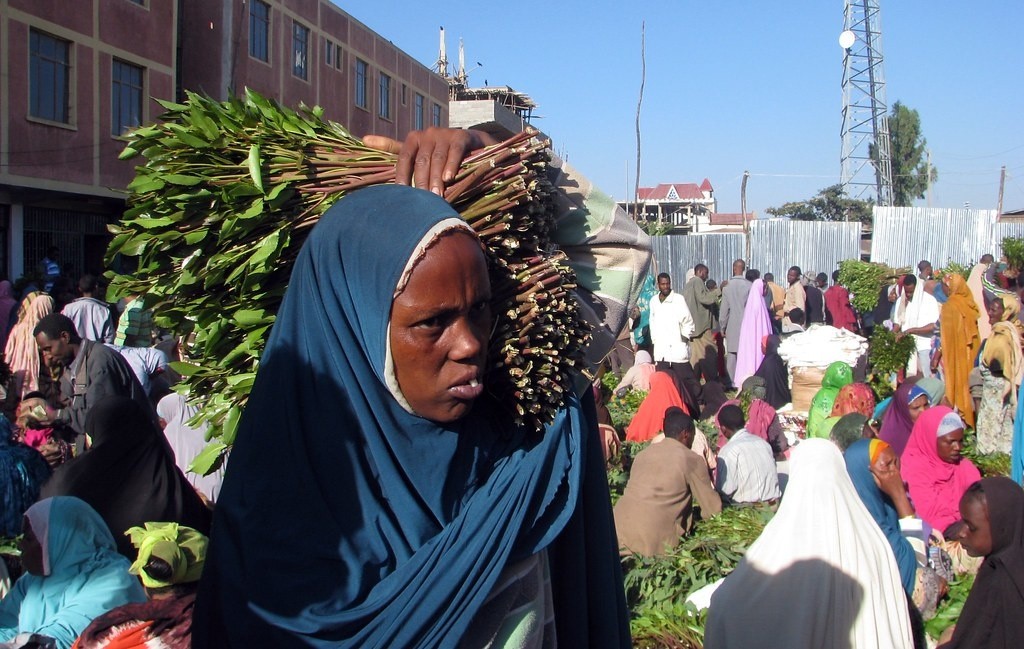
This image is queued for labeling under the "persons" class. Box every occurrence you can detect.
[2,241,246,649]
[187,120,639,649]
[587,240,1023,648]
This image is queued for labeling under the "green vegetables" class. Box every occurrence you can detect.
[81,90,1023,649]
